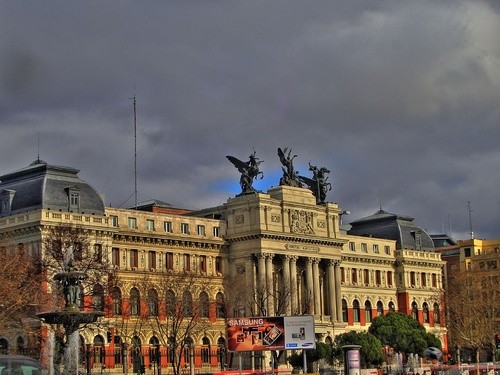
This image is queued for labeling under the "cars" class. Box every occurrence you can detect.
[0,354,63,375]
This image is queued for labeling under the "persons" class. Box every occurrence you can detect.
[224,145,332,205]
